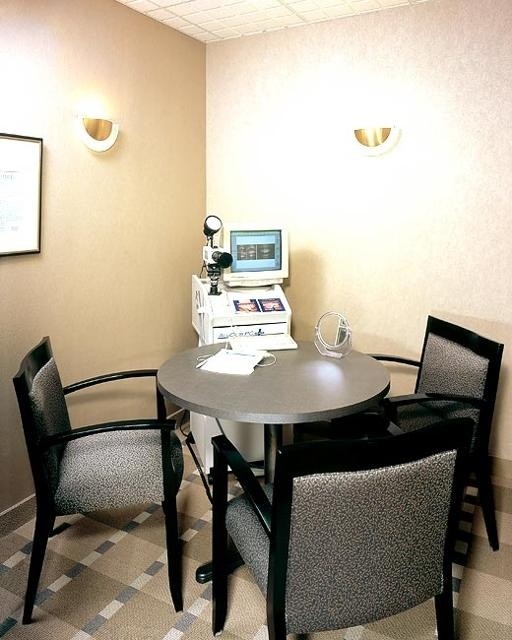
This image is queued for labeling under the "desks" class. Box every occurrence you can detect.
[158,335,390,583]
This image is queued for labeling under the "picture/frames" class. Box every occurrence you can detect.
[0,132,42,255]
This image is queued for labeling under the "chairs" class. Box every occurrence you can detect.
[13,335,184,624]
[341,314,503,554]
[212,414,476,638]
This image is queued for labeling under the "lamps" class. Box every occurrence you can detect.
[75,117,120,150]
[346,127,400,153]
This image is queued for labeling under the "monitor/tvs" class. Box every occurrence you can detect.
[220,225,289,294]
